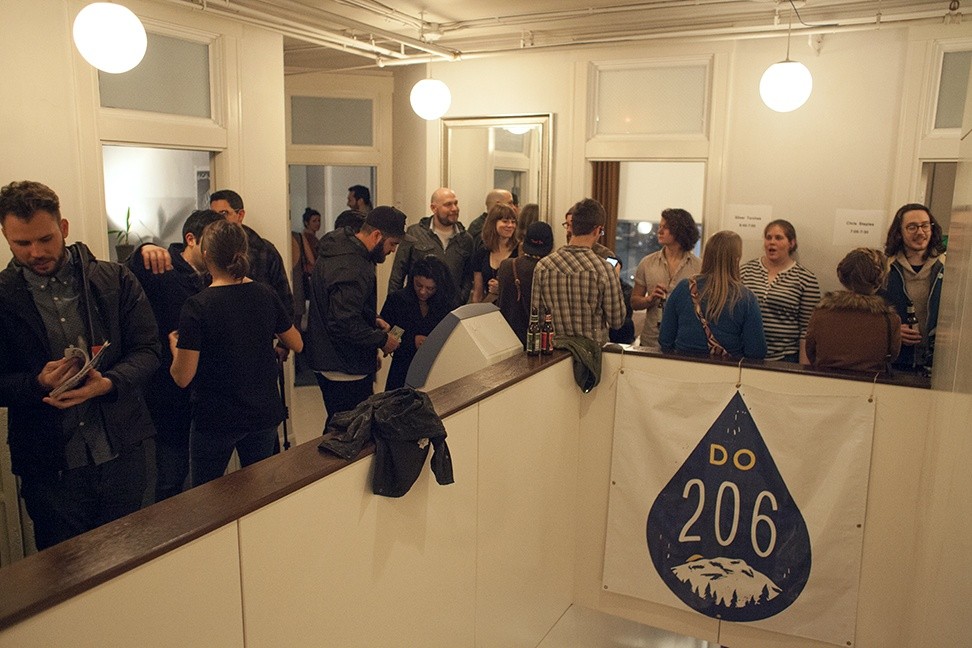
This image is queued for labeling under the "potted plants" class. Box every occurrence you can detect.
[108,206,142,262]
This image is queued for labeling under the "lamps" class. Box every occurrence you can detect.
[758,0,814,114]
[410,22,451,122]
[72,0,149,74]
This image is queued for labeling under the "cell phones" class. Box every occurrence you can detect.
[607,256,620,266]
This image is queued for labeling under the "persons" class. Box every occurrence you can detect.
[658,230,767,362]
[875,202,949,388]
[739,219,822,366]
[806,248,901,385]
[1,179,161,553]
[128,186,636,493]
[629,208,705,346]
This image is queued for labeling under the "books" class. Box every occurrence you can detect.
[46,340,111,399]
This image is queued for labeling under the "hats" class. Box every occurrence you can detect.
[522,222,554,257]
[365,206,419,244]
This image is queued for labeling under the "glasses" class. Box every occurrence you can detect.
[219,207,239,218]
[905,223,935,233]
[562,221,574,229]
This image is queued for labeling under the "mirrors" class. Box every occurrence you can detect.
[449,127,539,250]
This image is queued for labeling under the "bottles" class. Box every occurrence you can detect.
[527,308,540,356]
[542,307,554,355]
[906,300,920,335]
[657,290,666,327]
